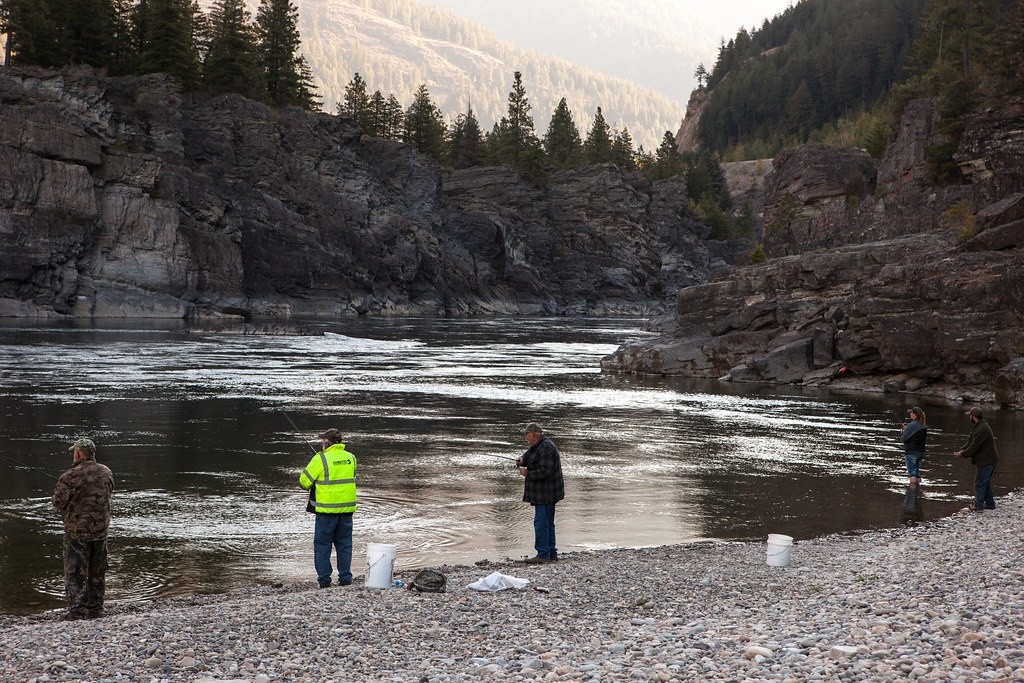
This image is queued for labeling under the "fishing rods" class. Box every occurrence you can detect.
[873,387,905,427]
[8,458,59,482]
[409,444,519,461]
[273,400,320,457]
[861,452,954,455]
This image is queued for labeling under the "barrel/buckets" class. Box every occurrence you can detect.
[765,533,794,567]
[364,542,397,589]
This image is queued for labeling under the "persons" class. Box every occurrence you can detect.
[954,406,999,512]
[900,406,928,483]
[52,439,115,621]
[516,423,565,563]
[298,428,357,588]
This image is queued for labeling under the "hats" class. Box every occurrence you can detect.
[965,406,984,415]
[69,438,96,452]
[521,422,543,436]
[908,407,922,413]
[318,428,342,440]
[407,568,447,593]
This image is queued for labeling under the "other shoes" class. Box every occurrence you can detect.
[969,504,983,510]
[60,610,105,620]
[525,556,558,565]
[320,583,331,588]
[983,503,995,509]
[339,581,352,586]
